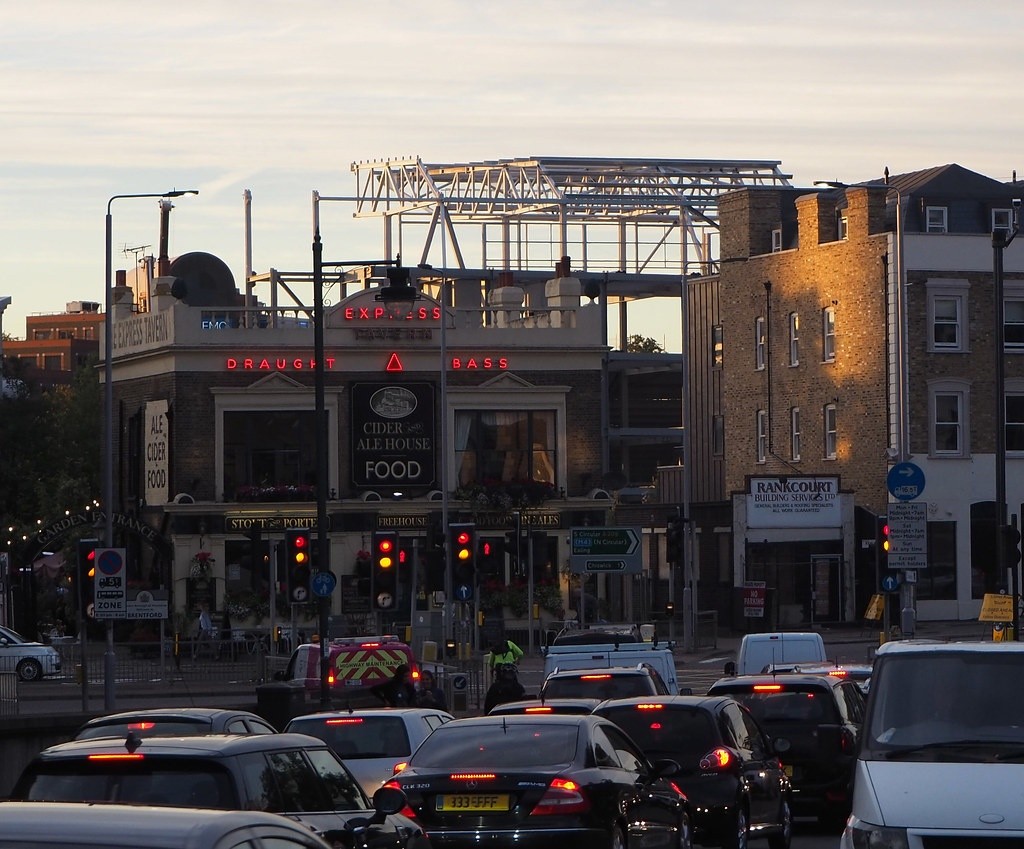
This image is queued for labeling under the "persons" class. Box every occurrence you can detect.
[484,663,526,716]
[382,664,417,708]
[417,670,447,711]
[195,604,219,661]
[489,640,523,671]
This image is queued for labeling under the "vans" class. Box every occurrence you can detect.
[838,637,1024,849]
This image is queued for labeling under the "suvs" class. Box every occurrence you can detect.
[7,729,433,849]
[588,692,795,849]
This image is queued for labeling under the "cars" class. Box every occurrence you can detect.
[70,707,282,744]
[0,624,62,683]
[276,705,456,810]
[0,798,331,849]
[255,633,422,731]
[382,713,692,849]
[479,617,876,830]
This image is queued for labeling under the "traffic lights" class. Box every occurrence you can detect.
[877,517,897,592]
[398,546,409,565]
[78,538,103,622]
[371,530,399,612]
[478,539,494,559]
[285,528,313,605]
[448,522,476,585]
[503,512,520,575]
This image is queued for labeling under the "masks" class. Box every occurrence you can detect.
[422,681,433,690]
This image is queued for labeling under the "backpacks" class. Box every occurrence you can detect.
[490,640,513,655]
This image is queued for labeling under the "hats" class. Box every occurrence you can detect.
[395,665,410,673]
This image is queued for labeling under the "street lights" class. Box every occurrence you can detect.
[100,188,197,718]
[991,196,1024,643]
[813,178,916,640]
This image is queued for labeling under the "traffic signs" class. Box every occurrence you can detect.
[886,461,926,501]
[569,524,643,574]
